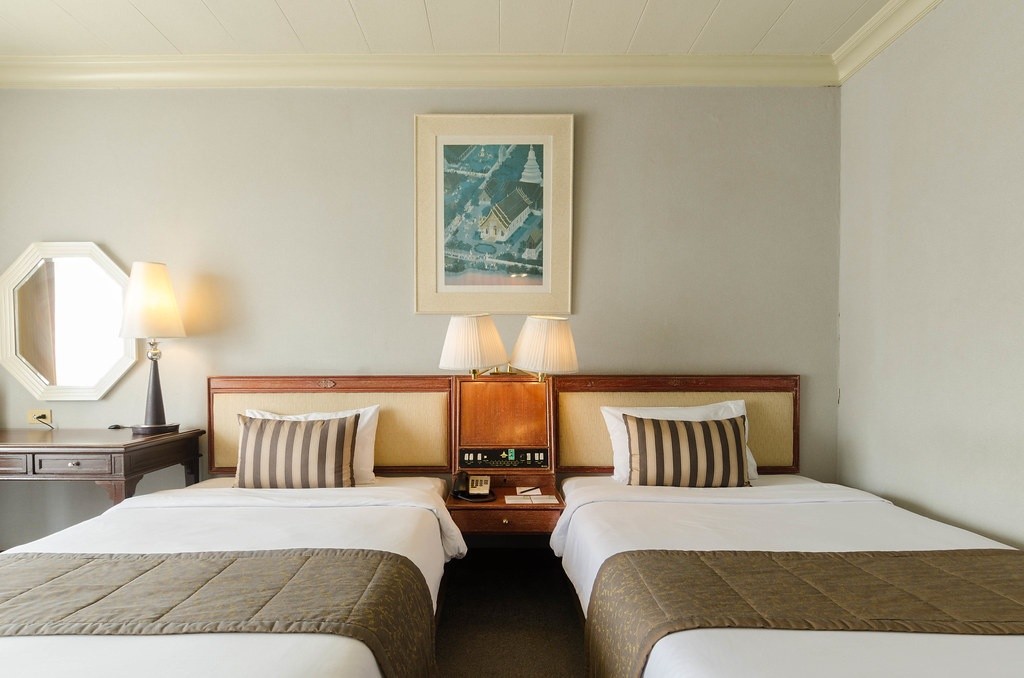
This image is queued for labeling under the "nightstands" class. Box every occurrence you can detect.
[443,480,566,534]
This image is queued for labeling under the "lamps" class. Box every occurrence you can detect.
[126,259,186,434]
[438,314,579,383]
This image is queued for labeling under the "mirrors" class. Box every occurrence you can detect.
[0,242,139,400]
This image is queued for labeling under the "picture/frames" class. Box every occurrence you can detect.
[414,113,573,318]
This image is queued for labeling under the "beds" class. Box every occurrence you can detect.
[551,372,1024,677]
[0,373,466,677]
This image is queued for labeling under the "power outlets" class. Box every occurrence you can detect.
[28,410,52,423]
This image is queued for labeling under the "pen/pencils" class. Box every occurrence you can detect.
[520,486,540,493]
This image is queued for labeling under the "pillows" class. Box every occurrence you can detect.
[234,412,361,488]
[601,400,758,485]
[244,404,380,484]
[623,413,754,488]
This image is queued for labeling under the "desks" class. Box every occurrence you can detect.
[1,425,207,506]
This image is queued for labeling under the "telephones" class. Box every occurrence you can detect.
[450,471,491,498]
